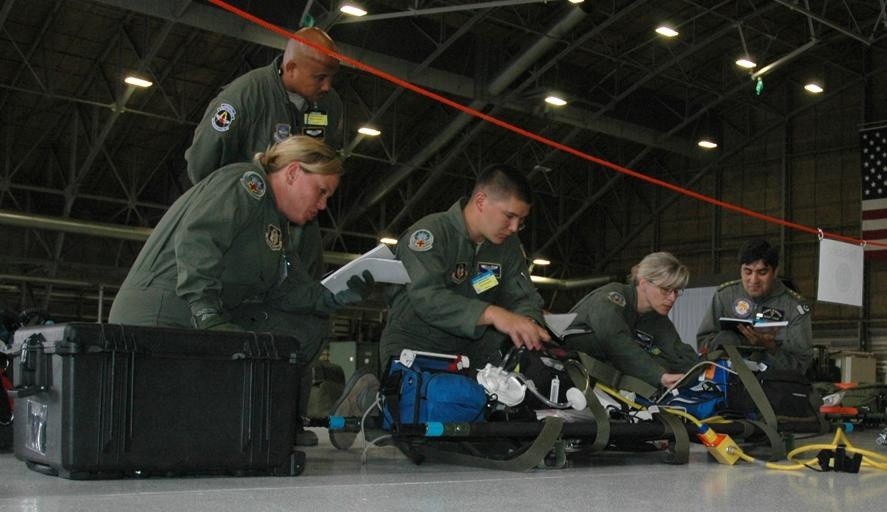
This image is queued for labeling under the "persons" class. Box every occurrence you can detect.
[105,133,375,376]
[326,160,551,450]
[696,237,814,442]
[184,25,344,447]
[560,251,728,453]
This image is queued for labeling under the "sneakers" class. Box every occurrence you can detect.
[295,428,318,447]
[329,368,381,451]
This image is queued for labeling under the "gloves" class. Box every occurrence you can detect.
[333,269,375,306]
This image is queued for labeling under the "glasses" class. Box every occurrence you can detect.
[646,278,684,297]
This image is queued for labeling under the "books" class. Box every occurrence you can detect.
[719,317,789,339]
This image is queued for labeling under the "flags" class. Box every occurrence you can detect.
[857,124,887,263]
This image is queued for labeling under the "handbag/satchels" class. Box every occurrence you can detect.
[669,356,729,420]
[381,348,487,430]
[730,367,813,416]
